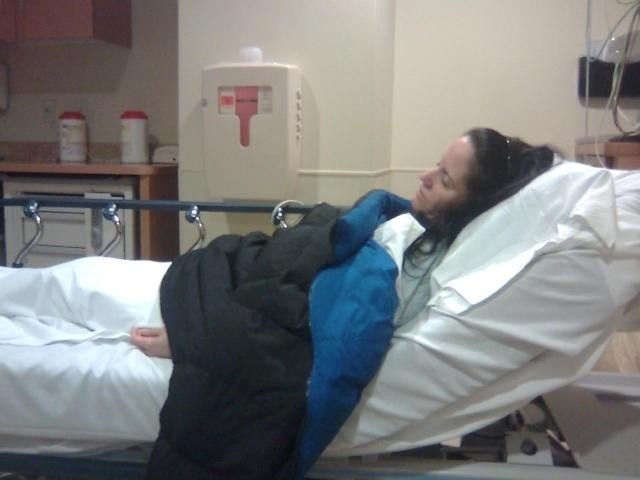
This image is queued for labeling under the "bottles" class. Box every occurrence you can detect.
[58,111,88,162]
[119,110,150,163]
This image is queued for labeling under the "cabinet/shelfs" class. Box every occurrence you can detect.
[0,0,133,46]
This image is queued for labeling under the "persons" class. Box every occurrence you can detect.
[123,124,563,358]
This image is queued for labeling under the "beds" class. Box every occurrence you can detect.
[2,150,638,480]
